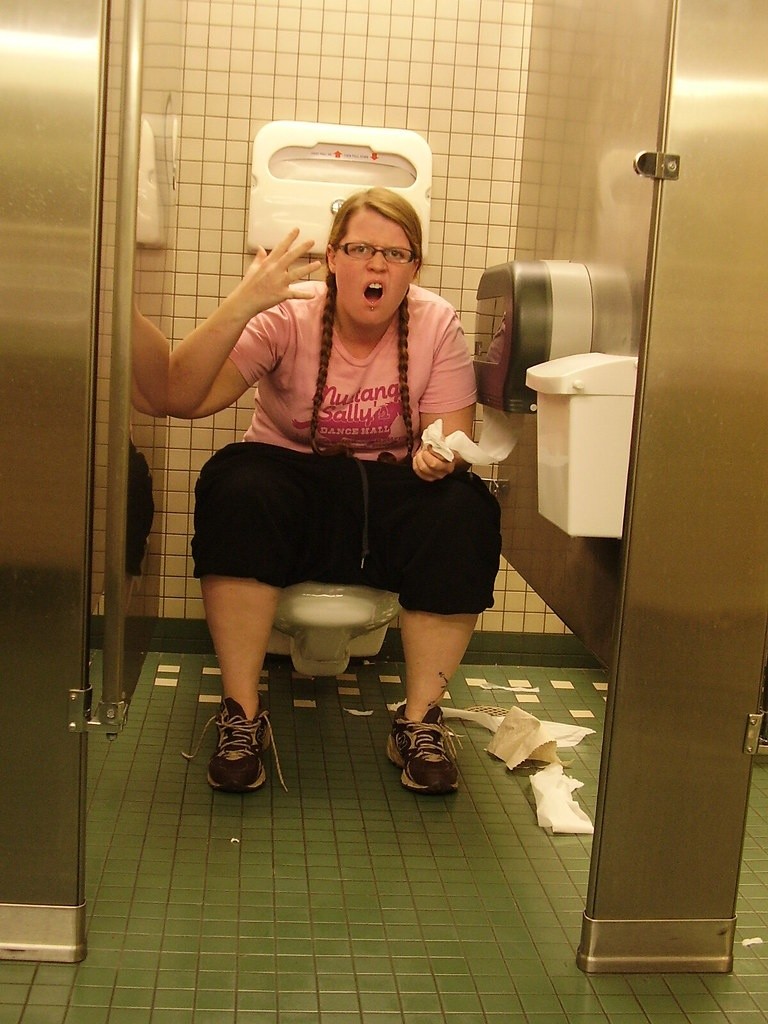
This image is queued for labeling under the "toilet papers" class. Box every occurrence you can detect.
[421,409,520,467]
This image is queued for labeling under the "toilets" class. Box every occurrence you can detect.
[273,581,399,676]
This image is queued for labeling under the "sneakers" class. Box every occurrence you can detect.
[180,697,288,793]
[386,704,459,794]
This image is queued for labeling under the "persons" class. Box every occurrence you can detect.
[168,188,508,794]
[124,309,174,614]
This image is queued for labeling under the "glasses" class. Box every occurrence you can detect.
[337,243,414,264]
